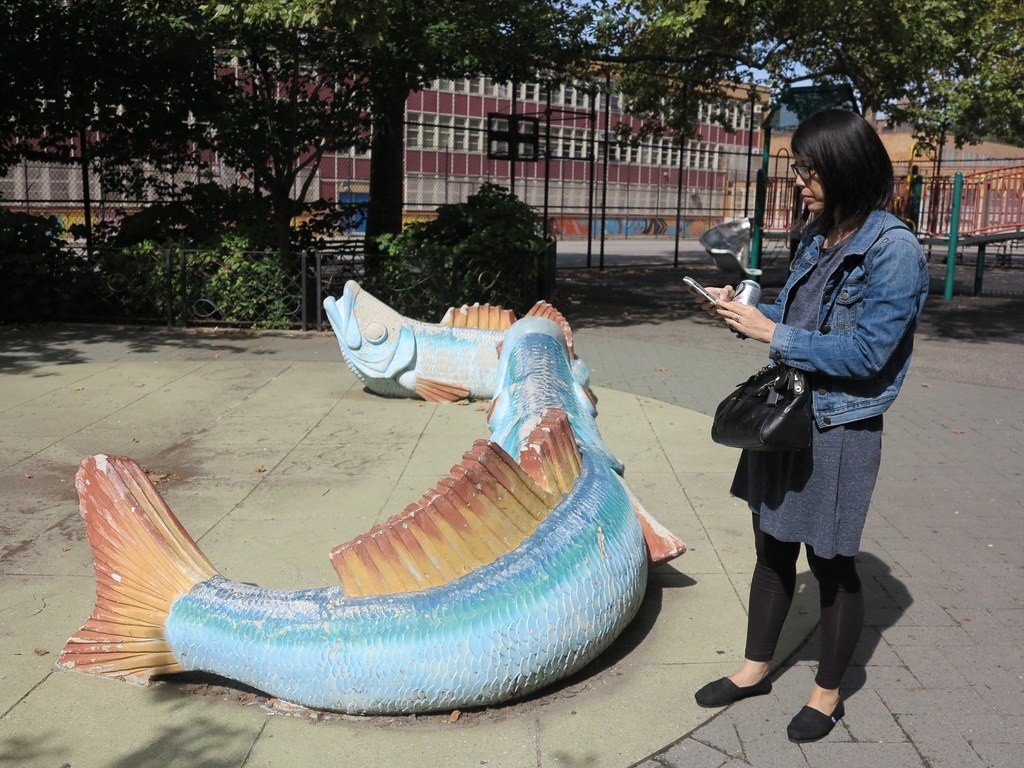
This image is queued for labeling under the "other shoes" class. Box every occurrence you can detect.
[786,688,845,743]
[694,671,772,707]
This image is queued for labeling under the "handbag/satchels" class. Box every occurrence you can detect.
[711,363,814,454]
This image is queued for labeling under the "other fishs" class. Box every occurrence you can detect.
[55,318,686,716]
[325,279,598,405]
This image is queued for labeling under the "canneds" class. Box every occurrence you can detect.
[726,280,761,331]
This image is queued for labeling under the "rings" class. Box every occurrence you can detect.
[735,314,740,322]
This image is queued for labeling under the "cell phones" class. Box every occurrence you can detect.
[682,276,726,312]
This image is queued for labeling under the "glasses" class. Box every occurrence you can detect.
[790,163,816,180]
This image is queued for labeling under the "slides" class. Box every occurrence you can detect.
[699,216,762,276]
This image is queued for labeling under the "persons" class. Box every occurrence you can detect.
[694,110,930,743]
